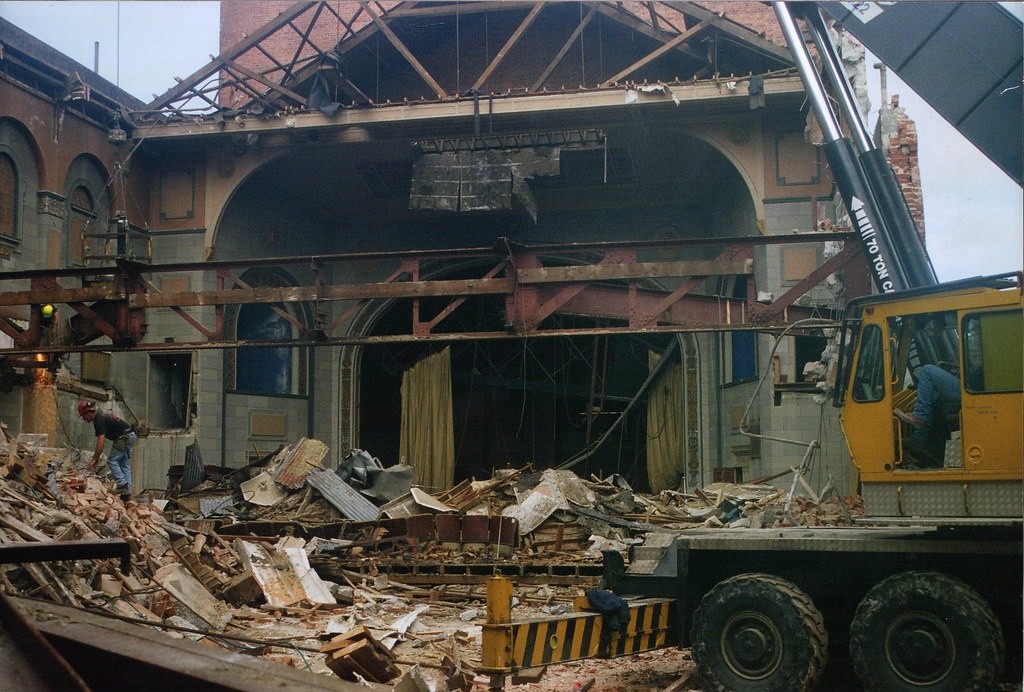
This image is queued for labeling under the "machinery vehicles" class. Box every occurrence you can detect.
[474,1,1024,692]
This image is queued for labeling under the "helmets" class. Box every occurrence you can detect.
[78,401,95,418]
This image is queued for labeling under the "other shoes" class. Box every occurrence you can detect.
[894,409,925,430]
[120,494,131,501]
[111,483,129,495]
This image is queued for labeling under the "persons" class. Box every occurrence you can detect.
[77,401,137,500]
[893,364,984,452]
